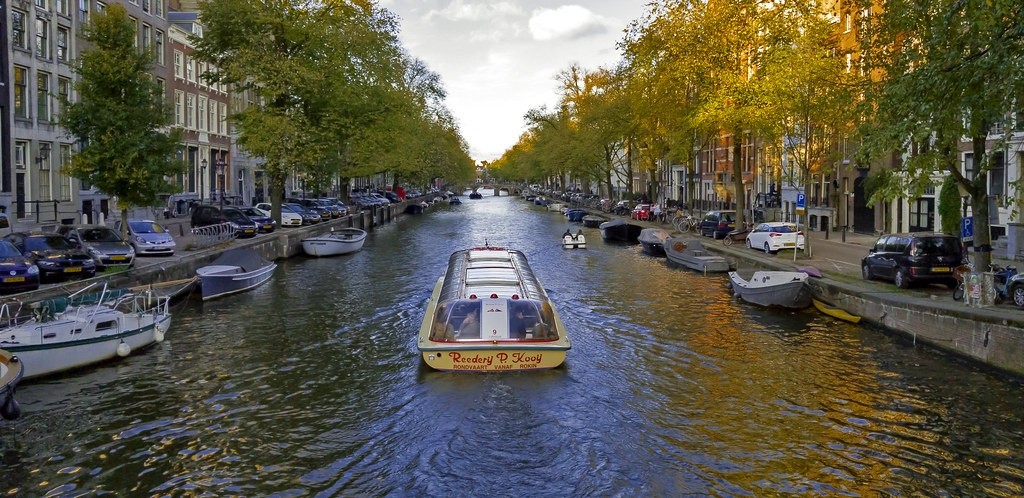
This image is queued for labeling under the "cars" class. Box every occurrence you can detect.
[1005,272,1024,309]
[630,204,653,221]
[0,236,40,294]
[520,184,640,228]
[2,230,97,283]
[56,224,135,272]
[745,222,805,255]
[112,218,176,255]
[228,186,454,228]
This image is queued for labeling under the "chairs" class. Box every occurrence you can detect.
[565,235,572,242]
[578,235,584,241]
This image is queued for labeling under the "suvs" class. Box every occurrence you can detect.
[860,230,964,289]
[190,204,259,239]
[699,210,746,240]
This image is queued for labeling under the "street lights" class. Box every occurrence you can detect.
[214,158,230,213]
[745,178,753,222]
[616,179,620,202]
[953,178,972,266]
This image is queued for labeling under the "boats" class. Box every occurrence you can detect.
[662,233,730,276]
[637,227,673,256]
[300,226,368,258]
[468,191,483,199]
[811,297,862,325]
[0,348,26,409]
[449,195,461,204]
[196,246,278,302]
[599,218,642,243]
[0,275,201,384]
[561,234,587,249]
[794,265,824,278]
[728,270,812,312]
[416,237,573,374]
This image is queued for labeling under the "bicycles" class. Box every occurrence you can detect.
[666,209,702,234]
[722,231,736,246]
[951,256,967,301]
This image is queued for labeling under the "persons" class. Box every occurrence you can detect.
[253,193,263,207]
[458,312,480,338]
[666,197,671,209]
[434,312,454,339]
[562,228,574,240]
[189,198,199,217]
[510,311,526,338]
[648,202,660,222]
[575,230,583,240]
[532,317,550,338]
